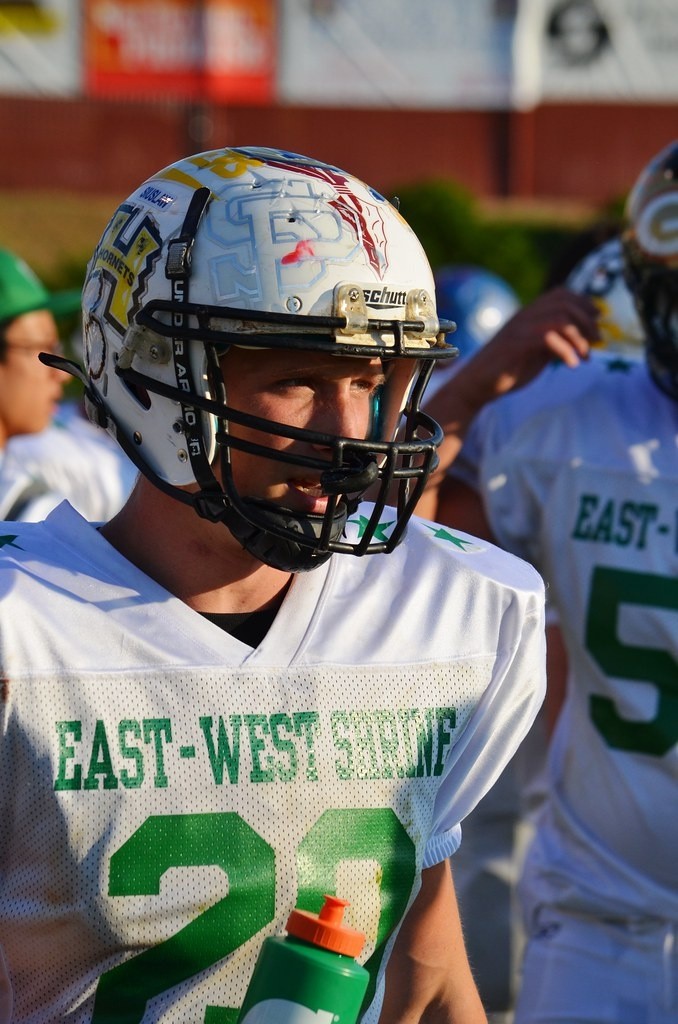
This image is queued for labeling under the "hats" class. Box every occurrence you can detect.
[0,246,82,319]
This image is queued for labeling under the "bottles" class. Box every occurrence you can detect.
[237,893,372,1024]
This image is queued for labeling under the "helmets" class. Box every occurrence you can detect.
[621,142,678,398]
[36,147,460,572]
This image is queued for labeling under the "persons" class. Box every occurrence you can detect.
[0,146,549,1024]
[2,228,650,447]
[368,128,677,1024]
